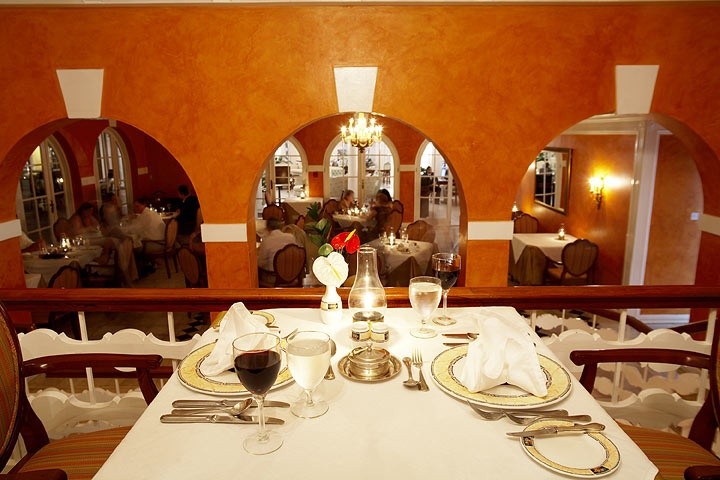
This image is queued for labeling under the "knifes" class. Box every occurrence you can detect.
[160,414,285,425]
[172,398,290,409]
[267,326,279,328]
[506,422,606,437]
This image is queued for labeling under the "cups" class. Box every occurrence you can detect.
[401,233,408,245]
[39,232,81,255]
[347,343,391,380]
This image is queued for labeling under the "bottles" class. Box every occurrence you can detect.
[390,227,395,245]
[557,223,565,239]
[348,247,388,323]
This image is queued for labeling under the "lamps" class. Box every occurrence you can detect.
[341,113,383,154]
[588,176,605,202]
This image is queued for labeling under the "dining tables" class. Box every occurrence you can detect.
[24,209,179,287]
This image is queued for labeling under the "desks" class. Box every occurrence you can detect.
[333,206,376,233]
[358,236,432,286]
[93,307,664,480]
[512,232,580,284]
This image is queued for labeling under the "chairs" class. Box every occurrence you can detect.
[48,266,79,289]
[53,218,72,241]
[514,213,539,233]
[569,305,720,480]
[178,246,204,287]
[547,239,599,286]
[138,218,178,278]
[84,237,135,288]
[260,192,434,287]
[0,302,163,480]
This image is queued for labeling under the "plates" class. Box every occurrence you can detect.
[176,341,295,396]
[248,309,276,327]
[430,343,572,410]
[520,417,621,478]
[338,355,402,382]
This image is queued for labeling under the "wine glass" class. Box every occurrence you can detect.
[408,276,443,340]
[232,332,284,455]
[149,203,171,215]
[431,252,462,326]
[285,330,331,420]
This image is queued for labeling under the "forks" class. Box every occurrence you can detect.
[411,347,429,391]
[504,411,591,425]
[467,400,568,421]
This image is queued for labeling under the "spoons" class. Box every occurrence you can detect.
[323,339,336,379]
[403,357,419,387]
[171,398,253,416]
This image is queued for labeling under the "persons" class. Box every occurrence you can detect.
[318,158,442,244]
[256,216,305,284]
[118,197,166,274]
[541,161,555,175]
[15,214,40,252]
[65,201,102,236]
[152,185,200,250]
[100,169,122,226]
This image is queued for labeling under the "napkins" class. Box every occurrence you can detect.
[199,301,266,376]
[456,318,549,397]
